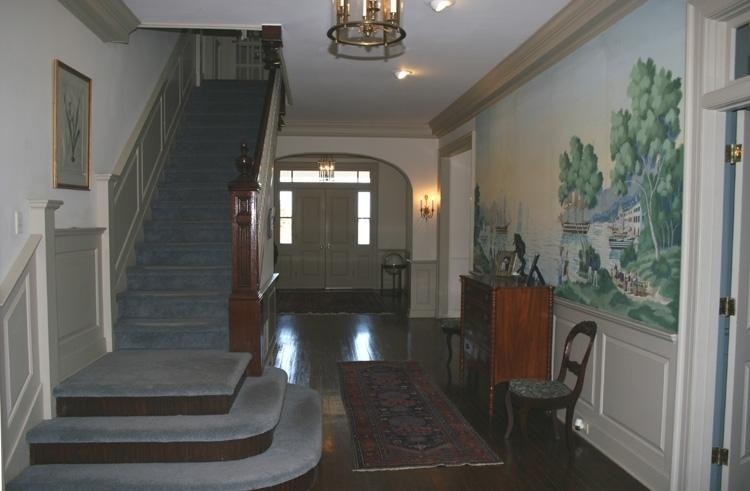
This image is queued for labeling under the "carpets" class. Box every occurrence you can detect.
[336,359,505,474]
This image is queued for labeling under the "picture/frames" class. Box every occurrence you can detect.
[51,58,94,193]
[473,2,687,335]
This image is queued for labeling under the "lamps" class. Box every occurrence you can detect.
[326,0,407,49]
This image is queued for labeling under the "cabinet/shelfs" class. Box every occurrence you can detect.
[458,275,556,418]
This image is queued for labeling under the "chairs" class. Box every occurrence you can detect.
[503,320,599,454]
